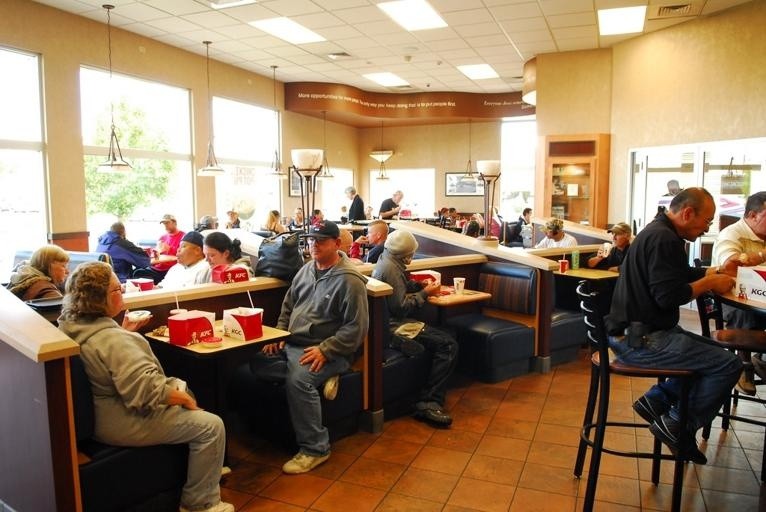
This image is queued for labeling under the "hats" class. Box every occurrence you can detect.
[608,222,632,234]
[160,214,176,223]
[383,229,419,259]
[180,232,204,247]
[546,219,563,231]
[200,215,218,225]
[301,220,340,239]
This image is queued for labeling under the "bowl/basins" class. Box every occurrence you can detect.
[128,309,151,322]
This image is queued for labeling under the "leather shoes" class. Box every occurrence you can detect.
[412,408,452,427]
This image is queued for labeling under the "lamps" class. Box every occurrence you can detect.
[97,3,135,177]
[266,64,289,181]
[369,150,394,163]
[461,120,475,184]
[474,160,503,239]
[196,40,225,177]
[374,119,388,183]
[316,108,336,180]
[288,149,328,253]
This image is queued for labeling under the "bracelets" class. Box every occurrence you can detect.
[759,252,765,263]
[714,266,720,274]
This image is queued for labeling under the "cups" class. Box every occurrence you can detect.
[558,260,568,275]
[169,309,187,315]
[152,248,161,261]
[452,276,466,295]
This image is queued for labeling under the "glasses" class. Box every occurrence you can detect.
[697,215,712,227]
[111,285,124,294]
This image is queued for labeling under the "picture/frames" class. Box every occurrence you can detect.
[444,170,484,198]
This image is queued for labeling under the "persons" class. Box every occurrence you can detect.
[345,186,366,220]
[55,260,235,512]
[370,228,460,425]
[378,190,404,218]
[708,189,766,398]
[339,220,389,263]
[535,218,577,248]
[602,187,744,465]
[9,245,70,300]
[441,207,532,242]
[97,211,250,290]
[664,180,683,196]
[265,207,324,234]
[585,222,631,273]
[247,219,372,477]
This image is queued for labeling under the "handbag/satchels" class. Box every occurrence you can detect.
[254,230,304,282]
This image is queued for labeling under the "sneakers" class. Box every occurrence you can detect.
[283,450,331,475]
[221,466,231,478]
[632,396,659,426]
[732,360,756,396]
[179,501,235,512]
[648,416,707,466]
